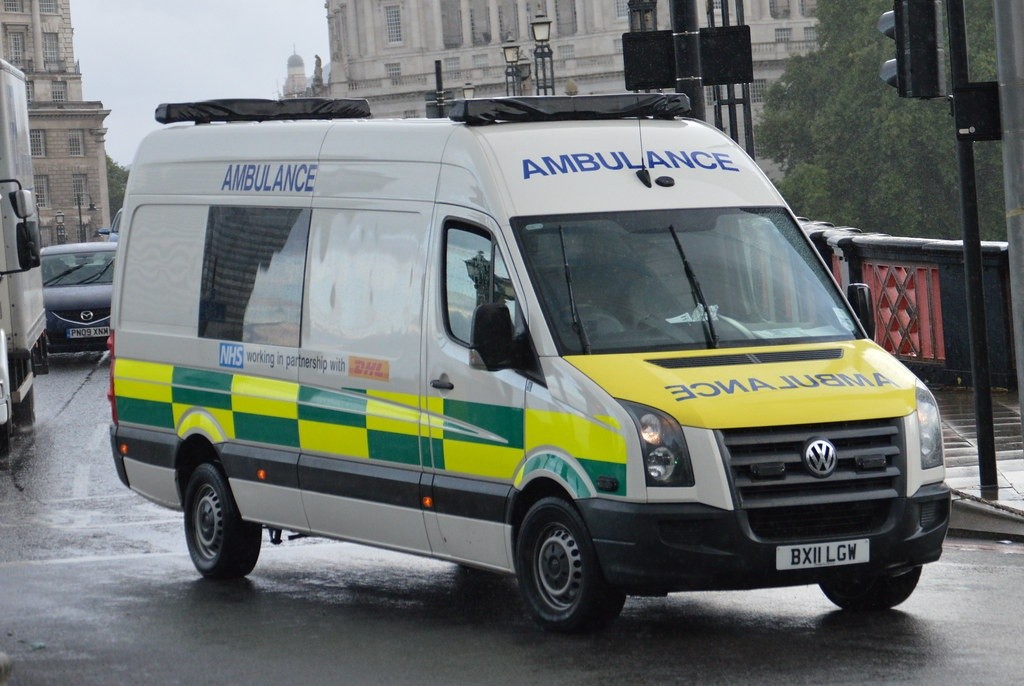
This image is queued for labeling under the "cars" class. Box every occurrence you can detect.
[37,242,121,353]
[98,208,124,242]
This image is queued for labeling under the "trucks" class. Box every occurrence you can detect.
[1,58,46,455]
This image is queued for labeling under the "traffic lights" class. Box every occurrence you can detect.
[877,0,947,100]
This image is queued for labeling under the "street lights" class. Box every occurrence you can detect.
[55,210,66,245]
[77,192,98,243]
[529,10,556,95]
[501,35,523,94]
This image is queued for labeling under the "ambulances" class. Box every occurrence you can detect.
[107,91,950,637]
